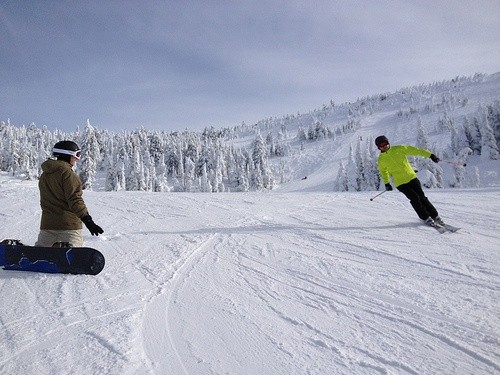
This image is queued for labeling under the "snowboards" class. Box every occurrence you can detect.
[0,242,105,275]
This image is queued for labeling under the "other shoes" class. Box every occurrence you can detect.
[425,216,446,229]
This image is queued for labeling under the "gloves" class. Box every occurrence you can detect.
[81,214,104,236]
[430,153,440,163]
[385,183,393,191]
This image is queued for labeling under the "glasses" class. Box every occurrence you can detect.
[379,143,388,148]
[72,150,82,160]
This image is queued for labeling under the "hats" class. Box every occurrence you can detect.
[374,136,389,146]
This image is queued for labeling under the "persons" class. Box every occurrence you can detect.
[34,140,104,247]
[374,135,444,228]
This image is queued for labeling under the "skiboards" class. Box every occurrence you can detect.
[419,219,446,234]
[438,221,461,232]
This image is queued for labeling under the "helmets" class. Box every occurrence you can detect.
[53,140,81,159]
